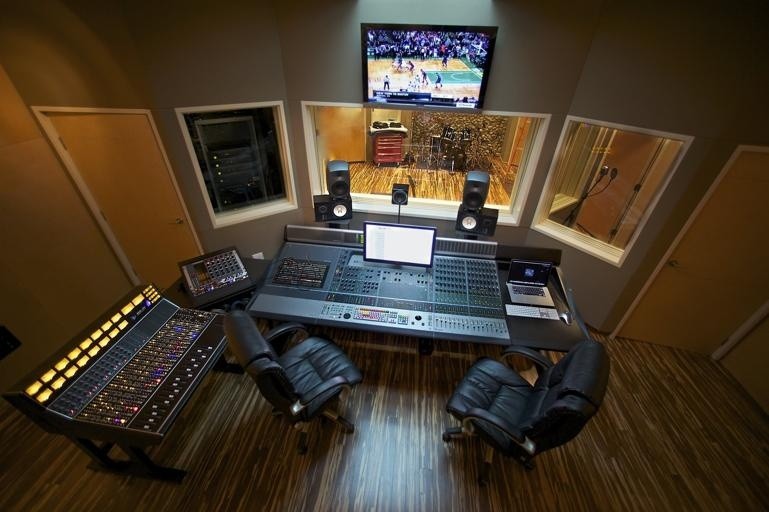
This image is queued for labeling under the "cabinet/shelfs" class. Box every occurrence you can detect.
[371,119,406,172]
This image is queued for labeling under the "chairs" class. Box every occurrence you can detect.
[441,337,612,486]
[226,307,369,455]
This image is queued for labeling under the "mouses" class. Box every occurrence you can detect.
[559,310,573,325]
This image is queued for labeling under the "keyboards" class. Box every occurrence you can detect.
[505,303,561,321]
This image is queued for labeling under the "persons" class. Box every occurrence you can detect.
[363,29,492,107]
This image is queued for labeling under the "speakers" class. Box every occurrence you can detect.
[391,184,409,206]
[313,195,352,222]
[461,171,490,210]
[326,160,350,196]
[455,204,499,236]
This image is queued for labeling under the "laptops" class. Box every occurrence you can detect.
[506,259,555,307]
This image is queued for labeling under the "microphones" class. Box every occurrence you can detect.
[601,166,608,175]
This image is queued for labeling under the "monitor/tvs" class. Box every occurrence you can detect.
[363,220,437,270]
[361,22,499,109]
